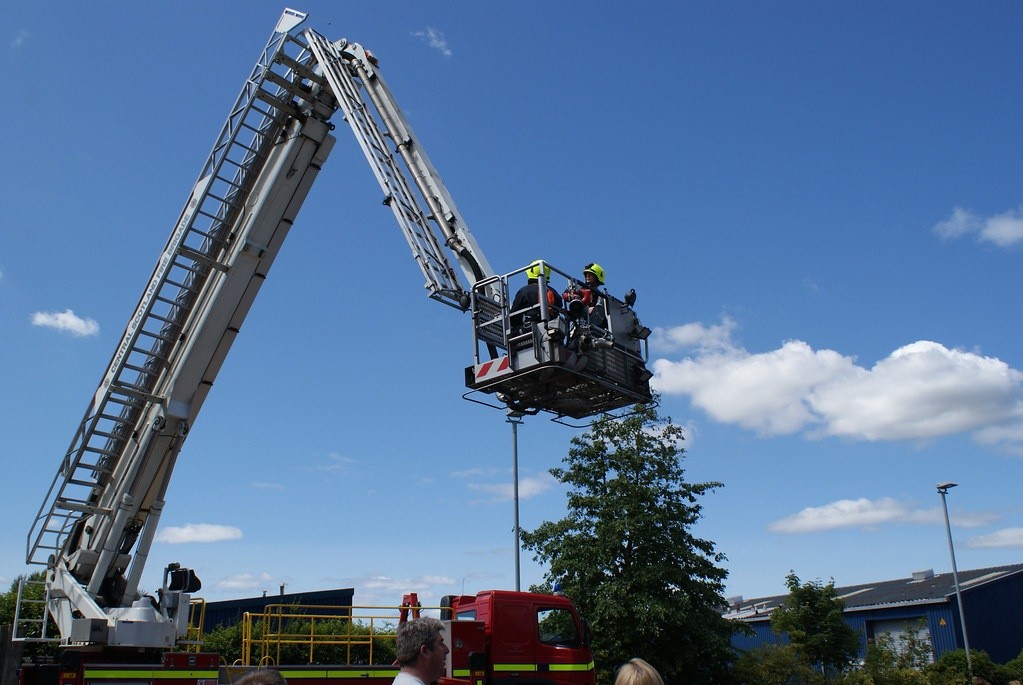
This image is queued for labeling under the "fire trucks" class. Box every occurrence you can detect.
[11,4,652,684]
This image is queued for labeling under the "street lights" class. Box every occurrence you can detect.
[935,480,976,685]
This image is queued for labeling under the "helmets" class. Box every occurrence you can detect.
[525,260,550,283]
[584,263,604,285]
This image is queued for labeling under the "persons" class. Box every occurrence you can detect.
[614,658,665,685]
[507,261,563,355]
[232,669,288,685]
[392,617,449,685]
[563,263,606,347]
